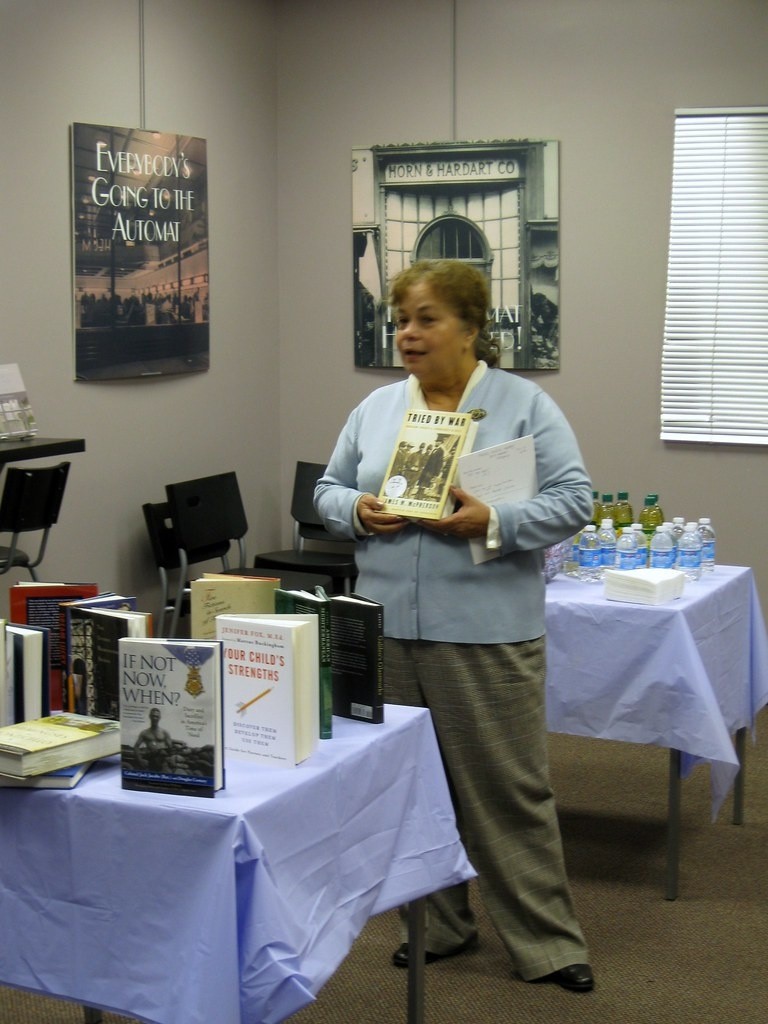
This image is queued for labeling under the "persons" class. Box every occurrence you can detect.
[312,260,596,991]
[80,287,209,321]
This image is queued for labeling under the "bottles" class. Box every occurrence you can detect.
[564,490,715,583]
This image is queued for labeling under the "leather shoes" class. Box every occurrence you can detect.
[393,941,443,968]
[558,962,595,992]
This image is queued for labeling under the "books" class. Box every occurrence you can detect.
[190,573,281,640]
[117,637,226,798]
[274,586,332,739]
[214,613,319,764]
[330,593,386,724]
[456,434,538,566]
[0,582,153,789]
[374,409,478,520]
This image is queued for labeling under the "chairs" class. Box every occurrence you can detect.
[141,462,359,638]
[0,461,72,583]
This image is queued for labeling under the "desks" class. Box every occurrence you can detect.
[539,564,768,900]
[0,702,479,1024]
[0,437,85,465]
